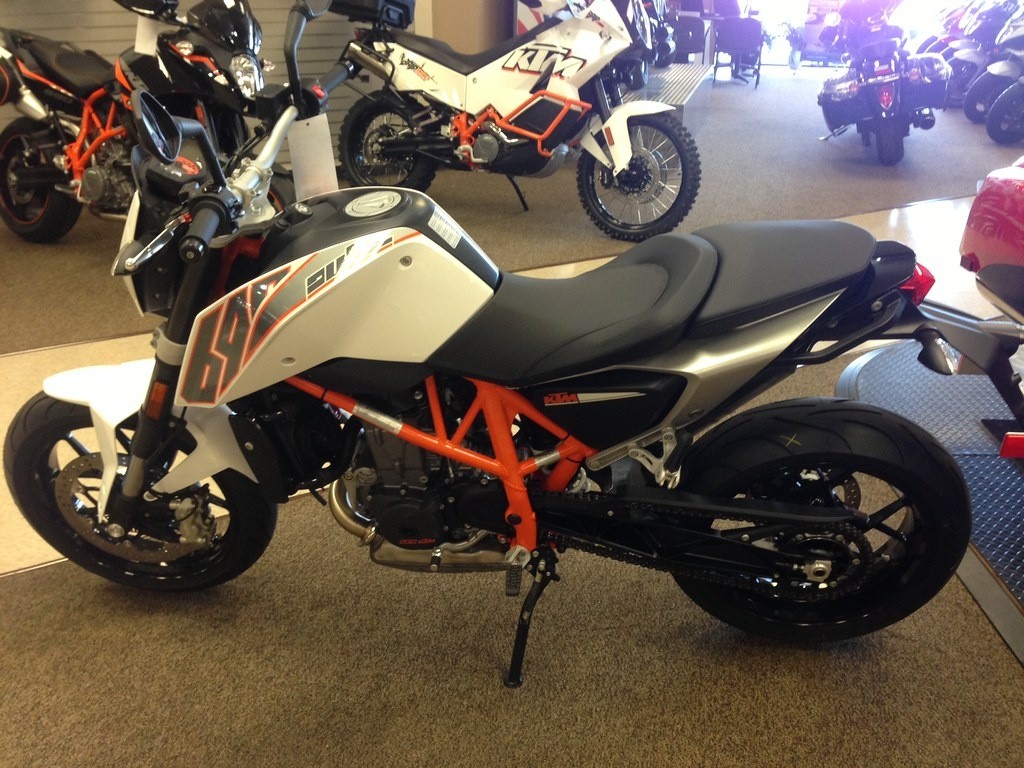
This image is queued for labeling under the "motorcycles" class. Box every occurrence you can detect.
[1,0,701,242]
[650,0,1024,169]
[1,0,973,690]
